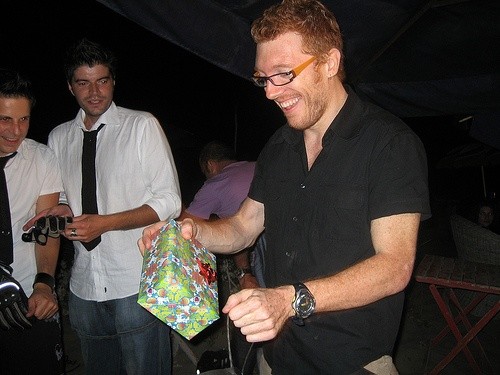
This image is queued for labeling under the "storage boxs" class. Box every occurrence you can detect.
[137,219,220,340]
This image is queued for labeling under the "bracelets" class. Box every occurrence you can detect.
[33,272,55,294]
[59,203,74,217]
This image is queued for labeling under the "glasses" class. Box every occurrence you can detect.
[251,57,316,88]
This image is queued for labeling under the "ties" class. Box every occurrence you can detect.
[79,123,107,252]
[0,151,18,267]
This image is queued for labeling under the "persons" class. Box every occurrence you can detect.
[474,201,497,229]
[22,46,182,375]
[138,0,431,375]
[174,141,256,375]
[0,69,64,375]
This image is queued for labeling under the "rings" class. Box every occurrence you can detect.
[71,229,77,237]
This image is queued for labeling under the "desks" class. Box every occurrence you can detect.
[414,253,500,375]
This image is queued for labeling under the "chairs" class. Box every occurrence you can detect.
[450,213,500,319]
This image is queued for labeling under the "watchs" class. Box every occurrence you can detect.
[291,283,316,325]
[235,268,252,279]
